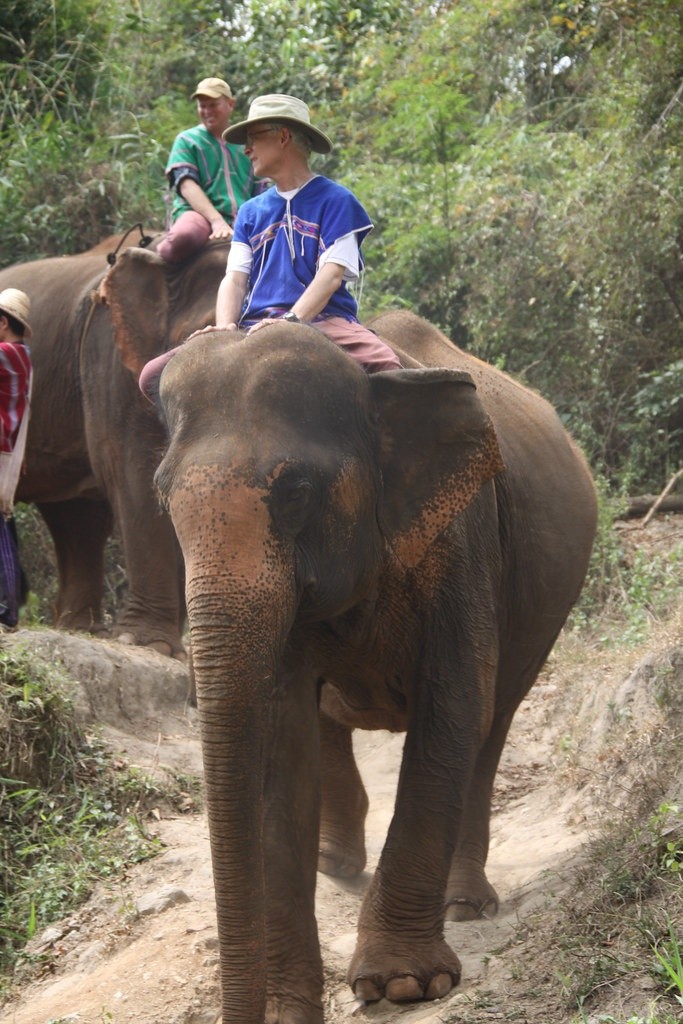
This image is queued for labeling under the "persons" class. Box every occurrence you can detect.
[137,94,406,409]
[156,78,279,266]
[1,286,33,632]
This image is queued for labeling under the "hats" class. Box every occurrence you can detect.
[0,288,32,339]
[221,94,333,155]
[190,77,231,100]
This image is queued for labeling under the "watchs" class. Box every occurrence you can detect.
[281,311,302,324]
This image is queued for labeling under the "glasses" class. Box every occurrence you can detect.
[245,129,292,147]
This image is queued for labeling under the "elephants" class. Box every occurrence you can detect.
[1,225,246,664]
[152,308,597,1024]
